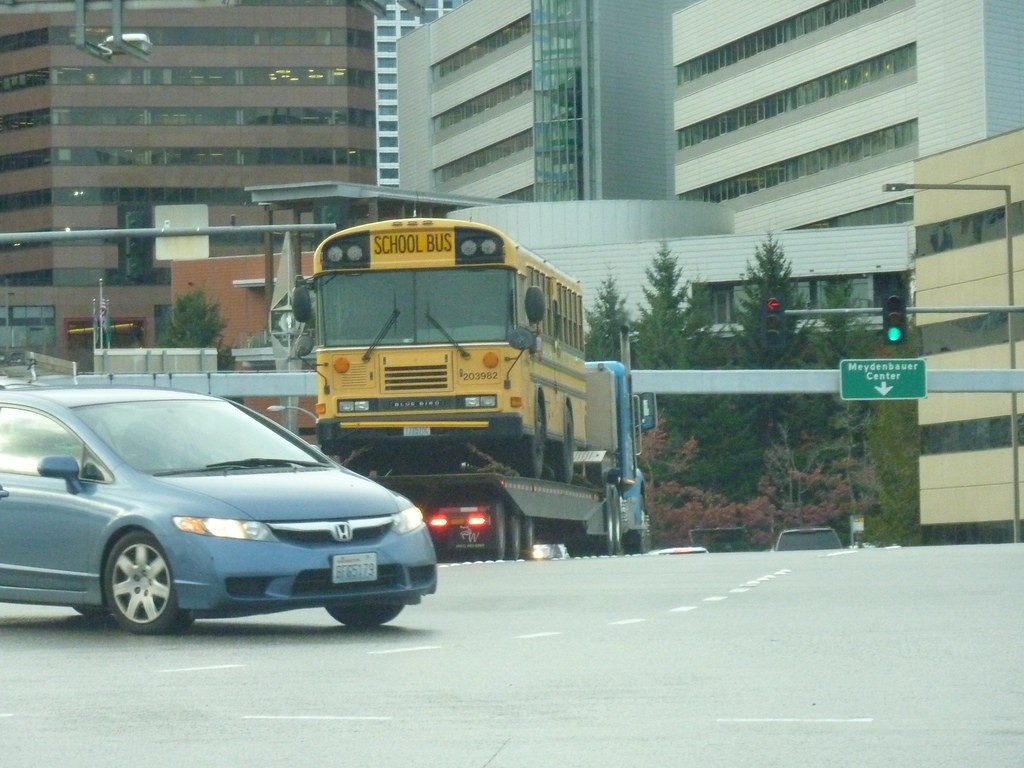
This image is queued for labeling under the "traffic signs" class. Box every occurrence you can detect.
[840,359,927,400]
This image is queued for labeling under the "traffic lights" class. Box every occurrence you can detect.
[764,292,786,348]
[882,290,907,346]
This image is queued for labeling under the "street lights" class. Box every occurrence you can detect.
[882,182,1021,546]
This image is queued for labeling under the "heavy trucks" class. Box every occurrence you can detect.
[364,360,658,561]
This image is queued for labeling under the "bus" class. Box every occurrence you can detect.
[290,219,588,484]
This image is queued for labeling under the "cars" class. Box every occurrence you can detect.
[775,528,843,551]
[0,387,438,634]
